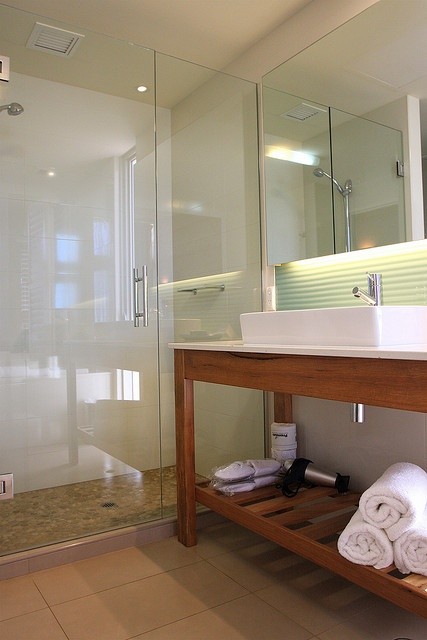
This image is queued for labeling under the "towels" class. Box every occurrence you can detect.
[358,461,427,543]
[337,507,394,570]
[395,514,427,577]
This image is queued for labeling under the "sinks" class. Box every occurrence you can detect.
[239,304,427,346]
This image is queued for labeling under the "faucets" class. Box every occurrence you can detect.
[350,287,375,305]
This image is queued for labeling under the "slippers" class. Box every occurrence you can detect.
[213,456,282,493]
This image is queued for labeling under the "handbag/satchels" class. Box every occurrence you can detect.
[270,422,299,450]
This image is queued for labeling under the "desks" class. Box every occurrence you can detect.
[173,348,427,621]
